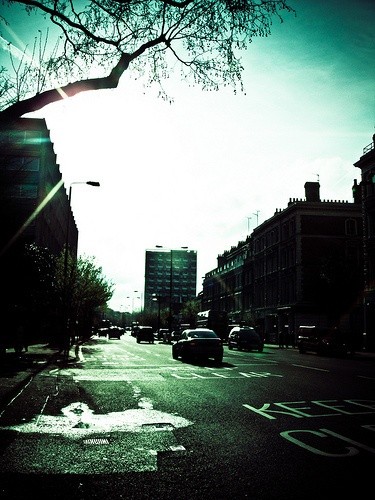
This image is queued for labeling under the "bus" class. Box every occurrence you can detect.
[195,310,230,334]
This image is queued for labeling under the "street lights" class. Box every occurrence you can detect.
[61,181,100,345]
[157,246,188,324]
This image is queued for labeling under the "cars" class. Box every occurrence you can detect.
[91,320,125,339]
[173,328,224,364]
[125,321,153,344]
[154,324,195,341]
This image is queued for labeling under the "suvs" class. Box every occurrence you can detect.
[228,327,264,351]
[297,325,351,356]
